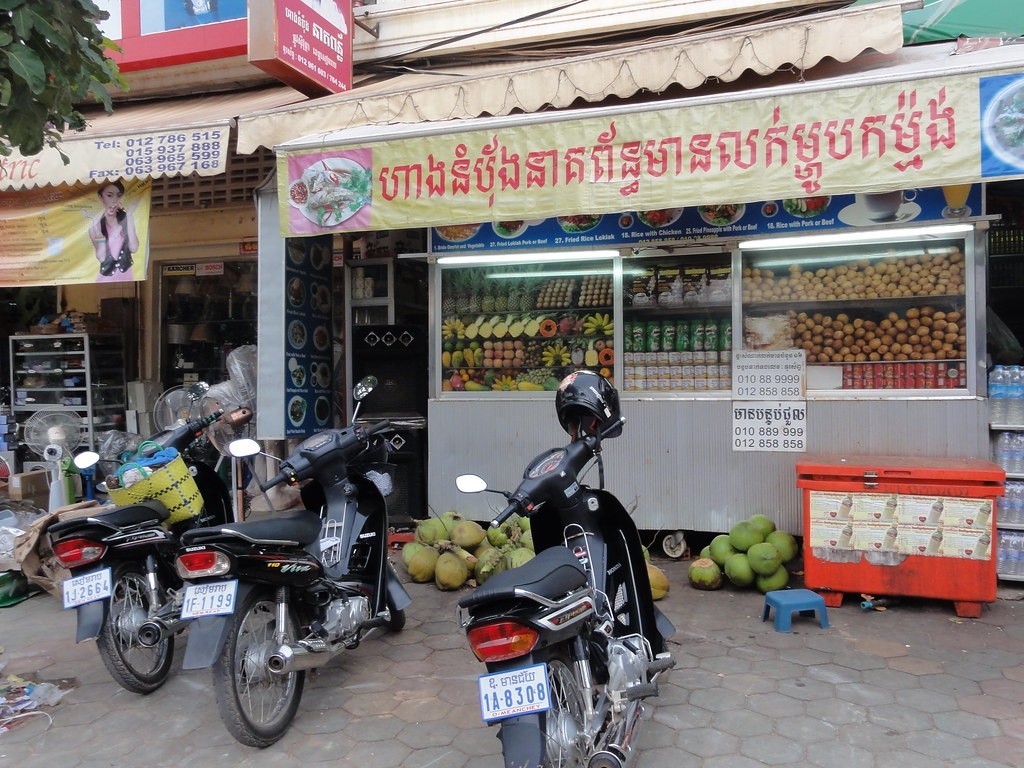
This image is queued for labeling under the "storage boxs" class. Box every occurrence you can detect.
[0,442,19,451]
[0,415,16,424]
[0,425,16,434]
[373,230,423,258]
[8,471,52,501]
[0,435,14,442]
[183,374,199,381]
[353,238,384,259]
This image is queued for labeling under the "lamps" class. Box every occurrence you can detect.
[427,247,633,265]
[168,324,188,370]
[191,324,215,352]
[727,219,990,250]
[174,275,199,303]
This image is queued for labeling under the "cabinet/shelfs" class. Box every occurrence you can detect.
[8,333,128,484]
[342,259,428,423]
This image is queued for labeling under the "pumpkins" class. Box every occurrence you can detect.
[540,319,557,337]
[599,348,614,365]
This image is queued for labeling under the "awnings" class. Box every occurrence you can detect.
[273,42,1024,239]
[232,2,904,157]
[0,75,377,190]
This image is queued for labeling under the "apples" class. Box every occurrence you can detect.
[600,368,612,378]
[483,340,526,368]
[459,368,475,381]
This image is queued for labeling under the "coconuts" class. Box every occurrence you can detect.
[402,511,669,601]
[688,514,798,594]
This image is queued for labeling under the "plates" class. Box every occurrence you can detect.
[619,212,634,230]
[696,203,746,226]
[782,196,832,218]
[286,237,331,428]
[492,222,528,240]
[301,158,371,227]
[434,223,485,243]
[761,201,778,217]
[837,202,921,227]
[556,214,604,234]
[636,208,684,227]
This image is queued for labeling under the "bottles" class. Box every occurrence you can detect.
[837,493,854,520]
[837,523,854,548]
[926,498,944,523]
[927,527,944,554]
[975,501,992,524]
[881,493,897,520]
[987,365,1024,575]
[882,522,899,547]
[974,529,991,556]
[988,220,1024,255]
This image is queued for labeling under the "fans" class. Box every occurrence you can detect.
[153,382,202,456]
[227,346,266,496]
[202,381,246,520]
[21,407,84,512]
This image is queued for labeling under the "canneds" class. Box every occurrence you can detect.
[624,351,733,390]
[843,362,966,389]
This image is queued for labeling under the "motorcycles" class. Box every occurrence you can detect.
[175,376,414,745]
[454,406,679,768]
[44,383,258,693]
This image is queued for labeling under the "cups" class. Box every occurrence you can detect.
[856,188,918,219]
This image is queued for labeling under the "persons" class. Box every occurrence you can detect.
[88,180,139,282]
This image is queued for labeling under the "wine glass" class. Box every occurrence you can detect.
[939,184,974,218]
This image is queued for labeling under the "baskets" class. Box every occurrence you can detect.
[101,441,204,531]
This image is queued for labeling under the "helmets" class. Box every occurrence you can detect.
[556,370,622,438]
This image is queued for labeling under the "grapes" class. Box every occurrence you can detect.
[515,368,555,386]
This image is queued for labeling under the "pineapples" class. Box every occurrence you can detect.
[443,268,543,315]
[441,368,453,391]
[465,312,559,338]
[585,338,599,366]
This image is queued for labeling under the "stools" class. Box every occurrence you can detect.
[763,590,828,630]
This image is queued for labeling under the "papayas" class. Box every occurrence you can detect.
[464,381,492,391]
[518,381,546,391]
[442,340,484,368]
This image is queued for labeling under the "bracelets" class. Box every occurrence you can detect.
[94,237,106,243]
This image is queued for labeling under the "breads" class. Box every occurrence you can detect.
[733,245,966,361]
[536,273,614,309]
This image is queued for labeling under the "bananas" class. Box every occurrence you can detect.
[583,313,614,336]
[541,343,571,366]
[442,315,466,339]
[491,374,517,391]
